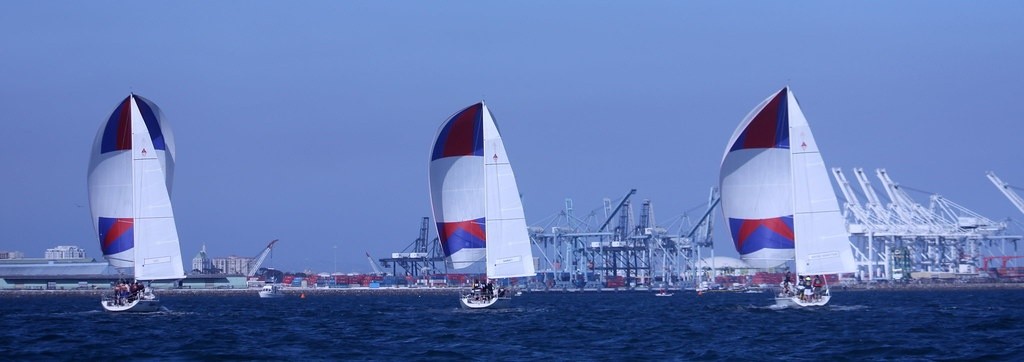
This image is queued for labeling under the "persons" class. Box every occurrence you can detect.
[782,271,792,295]
[813,275,822,302]
[797,276,805,300]
[114,281,145,306]
[474,279,494,302]
[803,276,814,303]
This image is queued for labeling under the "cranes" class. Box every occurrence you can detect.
[826,166,1023,284]
[246,239,280,282]
[523,184,723,289]
[365,249,388,277]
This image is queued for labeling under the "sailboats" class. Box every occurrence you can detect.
[85,93,184,313]
[717,86,858,310]
[427,101,535,313]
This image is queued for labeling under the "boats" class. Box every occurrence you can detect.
[258,279,285,300]
[655,290,675,297]
